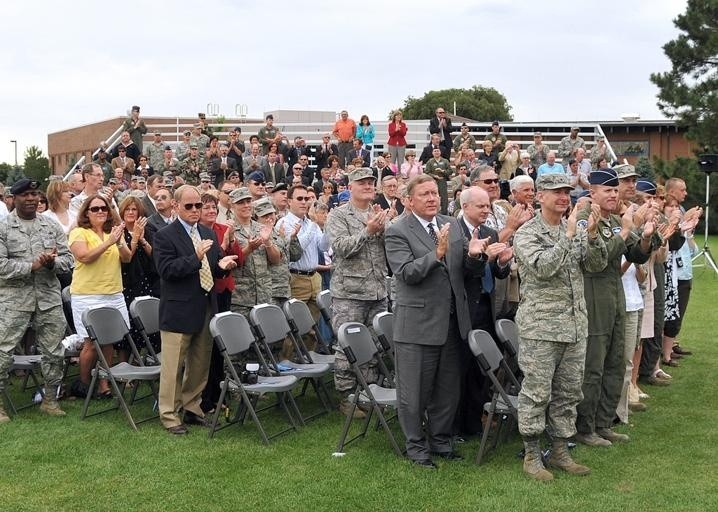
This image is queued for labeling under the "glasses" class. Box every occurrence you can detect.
[323,137,330,139]
[184,202,202,210]
[154,195,167,200]
[201,118,206,119]
[474,178,498,184]
[408,155,415,157]
[251,182,266,186]
[118,151,125,153]
[88,206,109,213]
[290,196,309,201]
[293,168,302,170]
[229,135,236,136]
[133,111,140,112]
[436,112,445,114]
[89,173,105,177]
[299,159,307,160]
[185,134,190,136]
[201,181,209,184]
[140,160,146,161]
[385,156,391,158]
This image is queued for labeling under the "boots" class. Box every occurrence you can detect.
[40,382,67,418]
[520,433,592,486]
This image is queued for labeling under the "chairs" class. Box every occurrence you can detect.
[342,397,373,419]
[336,321,431,456]
[82,305,161,431]
[209,311,297,440]
[495,319,524,442]
[372,311,431,431]
[248,303,328,428]
[467,329,523,467]
[0,290,339,409]
[577,333,695,450]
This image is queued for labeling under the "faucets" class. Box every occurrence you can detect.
[96,388,114,401]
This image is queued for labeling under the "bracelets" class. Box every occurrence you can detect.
[117,241,126,249]
[642,232,653,242]
[265,244,273,248]
[684,232,693,239]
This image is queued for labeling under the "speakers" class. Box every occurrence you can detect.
[40,382,67,418]
[520,433,592,486]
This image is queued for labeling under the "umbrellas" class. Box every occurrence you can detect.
[165,406,218,436]
[407,432,466,473]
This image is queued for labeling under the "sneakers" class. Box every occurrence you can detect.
[342,397,373,419]
[577,333,695,450]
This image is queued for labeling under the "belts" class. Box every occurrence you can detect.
[289,266,320,277]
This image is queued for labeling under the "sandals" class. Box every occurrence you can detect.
[96,388,114,401]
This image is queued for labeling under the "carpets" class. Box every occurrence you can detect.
[189,228,216,293]
[470,228,495,293]
[426,224,457,316]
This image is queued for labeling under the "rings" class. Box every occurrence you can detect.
[441,234,445,237]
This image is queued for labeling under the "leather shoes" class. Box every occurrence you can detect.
[407,432,466,473]
[165,406,218,436]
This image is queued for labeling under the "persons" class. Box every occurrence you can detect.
[122,105,149,156]
[3,120,704,426]
[193,112,213,140]
[428,107,453,161]
[510,171,604,485]
[575,167,661,447]
[2,178,75,421]
[257,115,279,156]
[387,110,408,175]
[332,110,358,168]
[325,168,395,418]
[382,172,494,468]
[356,114,376,168]
[152,184,239,434]
[66,193,132,401]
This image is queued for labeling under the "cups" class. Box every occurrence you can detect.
[184,202,202,210]
[88,206,109,213]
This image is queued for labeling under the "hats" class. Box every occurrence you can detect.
[248,171,265,182]
[536,172,575,191]
[183,130,191,135]
[137,177,145,184]
[109,178,117,184]
[194,124,202,129]
[199,113,205,118]
[253,198,277,217]
[131,176,137,181]
[190,144,198,149]
[613,164,641,179]
[133,107,140,110]
[348,168,377,185]
[636,180,656,195]
[153,131,161,135]
[571,126,580,131]
[595,135,604,141]
[590,168,619,187]
[164,146,171,150]
[271,183,287,193]
[228,171,239,180]
[164,180,172,187]
[266,182,274,188]
[461,122,466,126]
[10,180,41,194]
[434,145,439,150]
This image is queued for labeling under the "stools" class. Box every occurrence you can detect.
[289,266,320,277]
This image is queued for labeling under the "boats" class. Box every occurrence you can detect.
[699,154,718,172]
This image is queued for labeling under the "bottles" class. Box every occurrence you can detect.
[253,198,277,217]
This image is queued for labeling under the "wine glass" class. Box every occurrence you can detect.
[441,234,445,237]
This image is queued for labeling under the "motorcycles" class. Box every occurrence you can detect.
[691,172,718,274]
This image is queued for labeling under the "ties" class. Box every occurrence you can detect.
[189,228,216,293]
[326,144,328,151]
[470,228,495,293]
[426,224,457,316]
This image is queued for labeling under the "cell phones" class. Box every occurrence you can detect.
[676,257,683,267]
[241,363,260,384]
